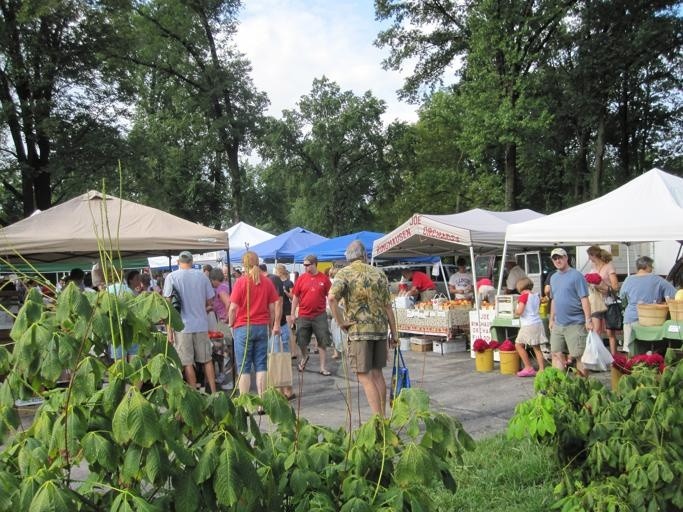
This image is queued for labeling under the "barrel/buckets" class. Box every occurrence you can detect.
[474,349,494,372]
[500,351,520,374]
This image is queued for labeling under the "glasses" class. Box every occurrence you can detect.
[304,264,312,266]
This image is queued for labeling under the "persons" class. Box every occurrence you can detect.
[328,241,399,419]
[545,269,556,314]
[620,256,678,353]
[587,246,621,356]
[504,256,527,294]
[162,251,216,396]
[228,252,283,415]
[0,266,166,396]
[291,256,333,376]
[204,268,236,389]
[402,269,438,303]
[330,296,347,359]
[548,248,593,378]
[274,265,295,353]
[257,264,281,352]
[514,277,548,378]
[448,258,474,302]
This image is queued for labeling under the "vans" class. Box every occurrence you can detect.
[374,264,463,299]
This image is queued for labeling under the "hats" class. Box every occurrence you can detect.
[551,249,569,257]
[179,251,193,262]
[68,268,84,279]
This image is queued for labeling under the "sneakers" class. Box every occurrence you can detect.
[517,368,536,377]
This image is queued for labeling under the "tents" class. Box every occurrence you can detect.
[194,221,276,264]
[148,253,181,269]
[31,210,41,216]
[504,169,682,241]
[3,258,161,272]
[297,228,386,260]
[226,228,330,259]
[1,190,229,254]
[372,207,548,257]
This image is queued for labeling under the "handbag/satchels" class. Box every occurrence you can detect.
[267,330,293,389]
[606,286,623,330]
[390,347,411,409]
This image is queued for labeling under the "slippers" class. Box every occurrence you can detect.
[298,356,309,371]
[320,370,331,375]
